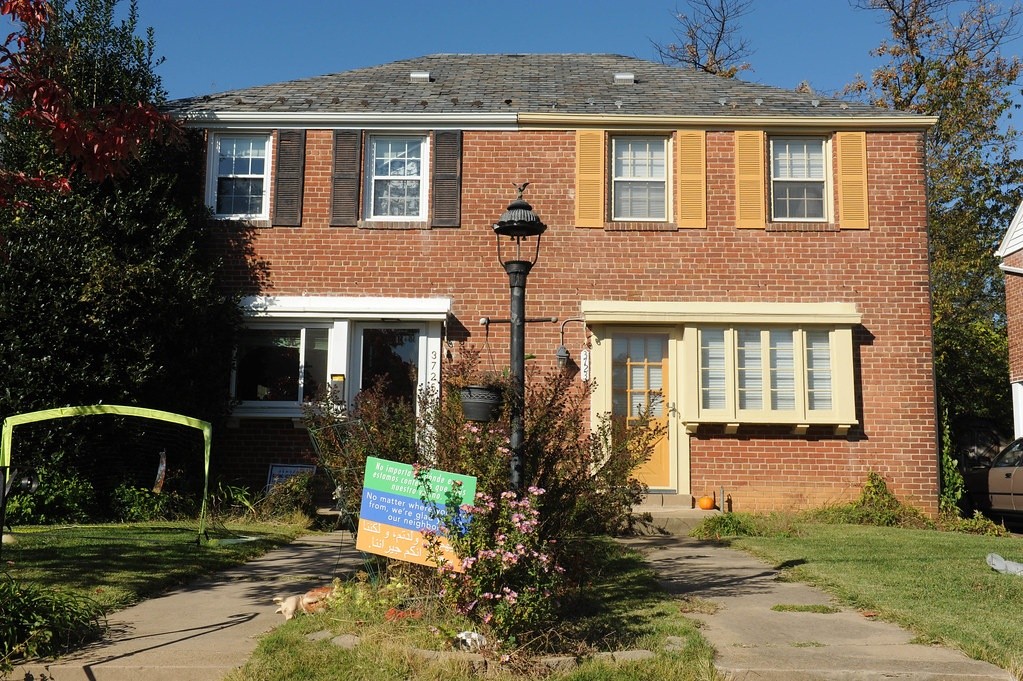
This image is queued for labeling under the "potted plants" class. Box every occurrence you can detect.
[461,371,505,424]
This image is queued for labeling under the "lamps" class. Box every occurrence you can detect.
[557,345,569,368]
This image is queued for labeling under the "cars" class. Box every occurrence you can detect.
[961,438,1023,526]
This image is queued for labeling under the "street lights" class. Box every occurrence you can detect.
[491,182,547,505]
